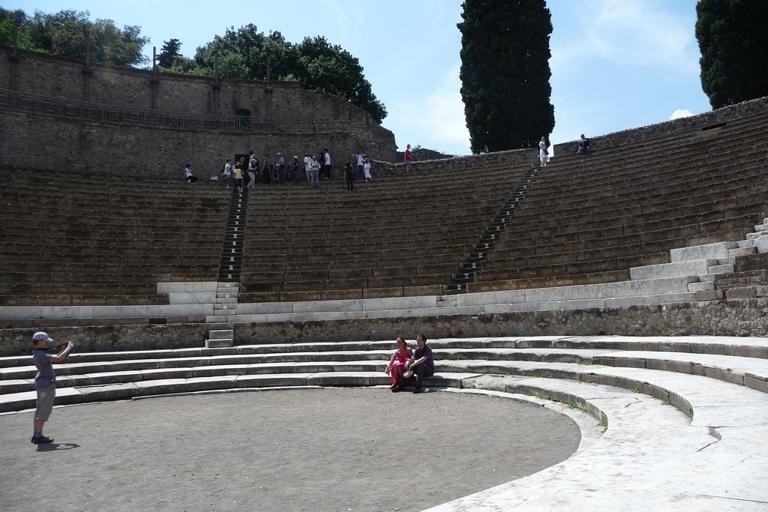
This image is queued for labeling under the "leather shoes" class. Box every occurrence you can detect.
[391,385,422,393]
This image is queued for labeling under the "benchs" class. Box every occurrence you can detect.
[2,111,768,309]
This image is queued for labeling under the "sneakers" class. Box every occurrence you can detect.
[32,436,54,444]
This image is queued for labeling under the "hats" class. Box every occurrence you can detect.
[32,331,54,342]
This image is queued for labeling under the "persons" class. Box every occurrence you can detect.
[385,336,414,392]
[221,148,373,191]
[183,163,197,184]
[398,335,434,394]
[31,332,74,444]
[539,136,549,168]
[404,144,413,174]
[576,134,592,155]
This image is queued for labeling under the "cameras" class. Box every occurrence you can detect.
[65,342,68,347]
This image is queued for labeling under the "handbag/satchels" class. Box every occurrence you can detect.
[402,370,414,385]
